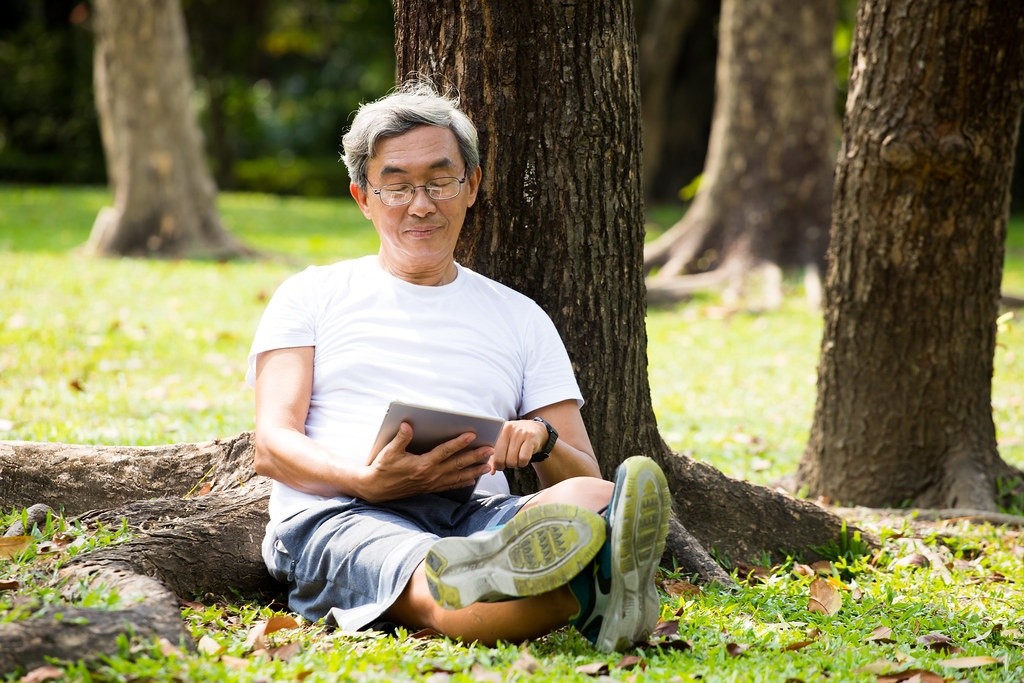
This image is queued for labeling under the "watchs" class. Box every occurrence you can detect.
[530,415,560,462]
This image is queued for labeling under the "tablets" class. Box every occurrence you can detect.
[366,401,505,504]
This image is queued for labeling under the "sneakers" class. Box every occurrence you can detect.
[423,503,608,611]
[569,454,671,652]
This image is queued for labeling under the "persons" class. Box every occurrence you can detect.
[247,82,671,656]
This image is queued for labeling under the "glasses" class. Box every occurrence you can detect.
[361,159,468,207]
